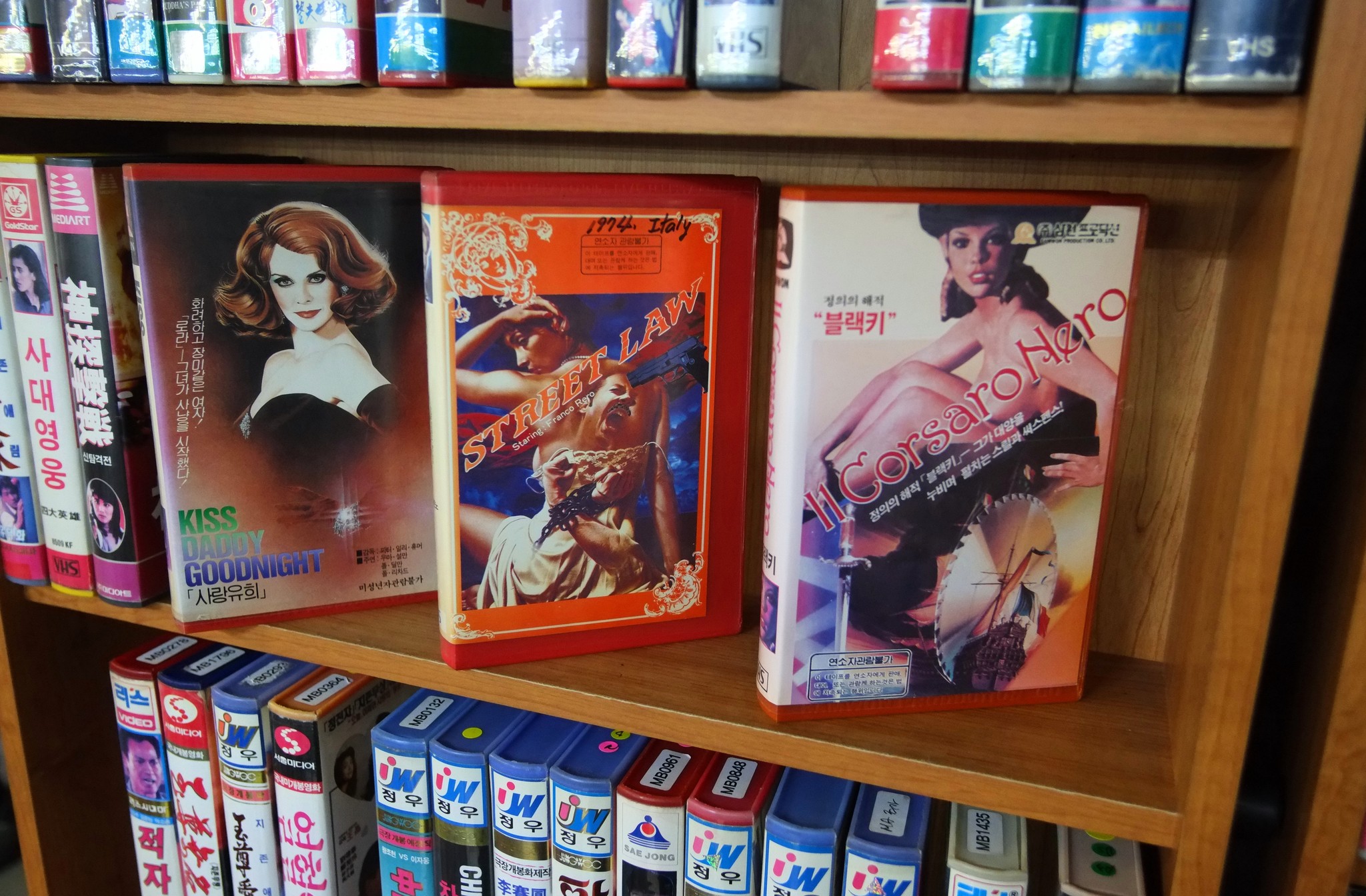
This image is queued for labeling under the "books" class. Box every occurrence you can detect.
[1,0,1315,896]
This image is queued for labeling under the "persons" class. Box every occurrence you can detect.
[9,242,52,316]
[332,747,360,795]
[117,725,164,802]
[801,205,1117,526]
[212,202,403,487]
[453,294,681,608]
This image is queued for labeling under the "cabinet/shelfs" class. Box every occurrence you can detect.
[0,0,1366,896]
